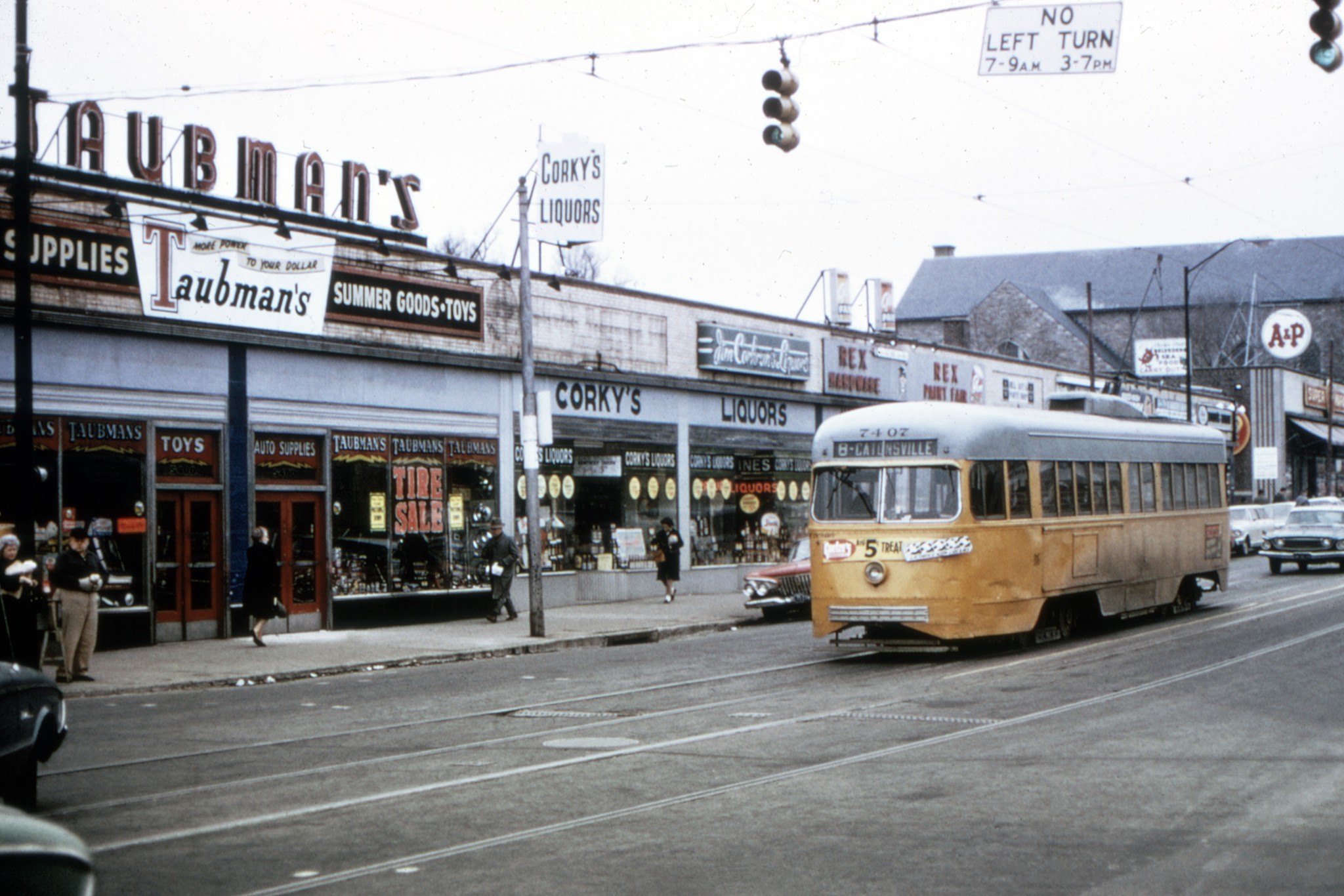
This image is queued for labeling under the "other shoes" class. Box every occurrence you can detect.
[73,675,94,681]
[506,613,517,621]
[670,588,676,601]
[254,639,265,647]
[486,615,497,622]
[249,629,260,643]
[664,594,672,603]
[56,676,67,683]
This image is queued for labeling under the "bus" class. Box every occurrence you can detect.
[807,401,1228,657]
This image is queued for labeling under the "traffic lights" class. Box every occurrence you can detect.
[761,67,802,153]
[1309,0,1343,73]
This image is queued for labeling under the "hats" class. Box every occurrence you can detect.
[489,517,505,529]
[661,517,675,526]
[70,527,87,541]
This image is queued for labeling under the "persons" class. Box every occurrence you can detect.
[1,532,44,670]
[651,516,684,602]
[48,526,105,682]
[1273,487,1288,503]
[942,471,983,517]
[243,524,280,646]
[481,518,521,623]
[1254,488,1269,505]
[1295,488,1310,507]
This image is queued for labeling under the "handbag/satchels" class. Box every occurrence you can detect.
[651,549,665,565]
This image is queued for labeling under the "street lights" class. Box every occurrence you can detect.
[1183,239,1274,423]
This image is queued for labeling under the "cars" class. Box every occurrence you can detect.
[1262,500,1294,530]
[1226,503,1272,557]
[1257,504,1344,575]
[742,536,814,622]
